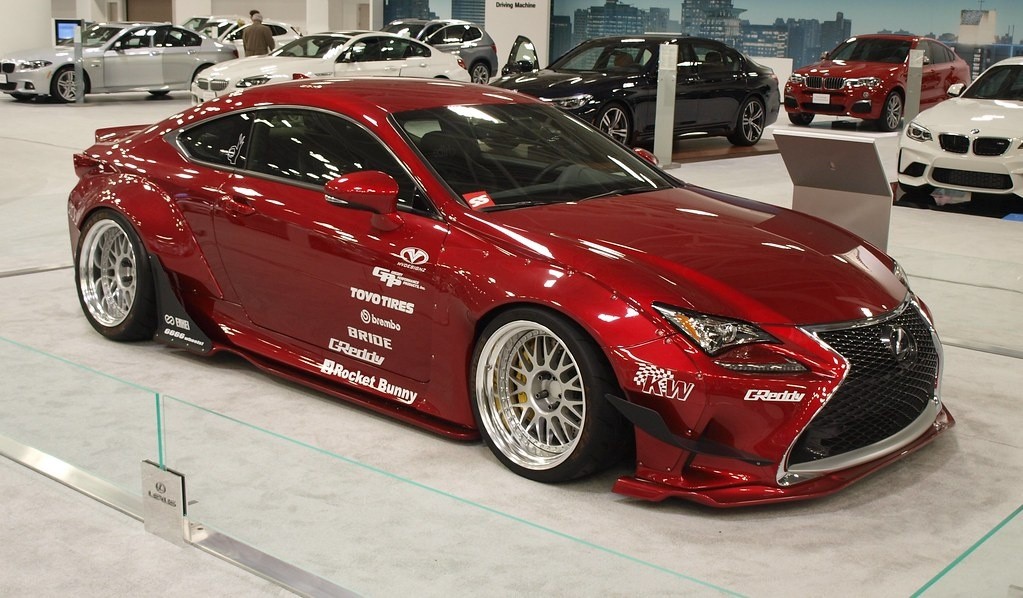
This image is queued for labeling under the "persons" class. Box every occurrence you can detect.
[242,9,275,57]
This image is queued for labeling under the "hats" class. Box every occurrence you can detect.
[252,13,262,22]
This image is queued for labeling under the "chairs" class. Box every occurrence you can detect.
[696,52,725,75]
[420,131,501,197]
[611,52,633,72]
[297,114,359,188]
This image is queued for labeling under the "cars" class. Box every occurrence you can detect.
[189,29,472,103]
[492,32,781,148]
[784,34,971,131]
[895,55,1023,199]
[379,18,499,84]
[183,16,304,56]
[0,20,239,103]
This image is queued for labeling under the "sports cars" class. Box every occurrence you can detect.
[65,76,956,510]
